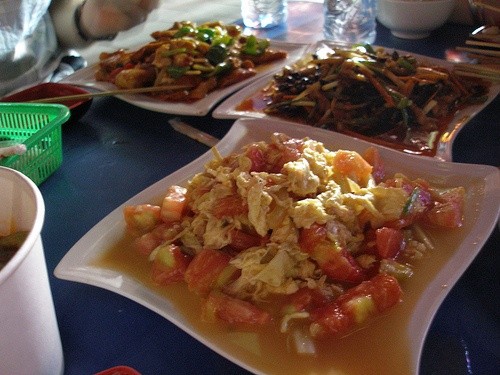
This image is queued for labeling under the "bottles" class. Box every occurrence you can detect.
[241,0,287,29]
[323,0,377,46]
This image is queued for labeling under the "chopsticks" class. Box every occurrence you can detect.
[456,41,500,57]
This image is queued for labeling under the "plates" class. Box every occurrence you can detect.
[54,116,500,375]
[212,38,500,163]
[58,42,313,116]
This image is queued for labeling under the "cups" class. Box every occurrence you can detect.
[0,166,65,375]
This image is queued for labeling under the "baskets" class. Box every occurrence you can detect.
[0,101,72,187]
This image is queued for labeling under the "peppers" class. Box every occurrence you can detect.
[160,25,270,79]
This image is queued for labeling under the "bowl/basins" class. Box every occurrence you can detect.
[0,82,92,120]
[375,0,455,38]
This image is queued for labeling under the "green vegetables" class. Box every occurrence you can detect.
[268,43,492,141]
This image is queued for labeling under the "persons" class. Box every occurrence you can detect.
[0,0,160,99]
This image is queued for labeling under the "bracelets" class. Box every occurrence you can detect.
[74,0,117,43]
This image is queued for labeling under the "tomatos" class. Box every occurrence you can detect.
[123,133,465,337]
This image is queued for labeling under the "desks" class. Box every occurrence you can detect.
[39,0,500,375]
